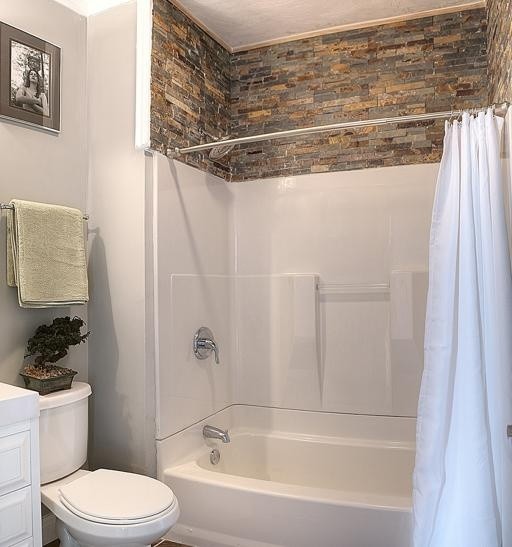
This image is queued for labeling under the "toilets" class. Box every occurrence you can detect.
[38,380,181,547]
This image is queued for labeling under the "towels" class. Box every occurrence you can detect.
[4,198,90,311]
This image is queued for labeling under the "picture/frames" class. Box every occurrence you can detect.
[0,20,63,137]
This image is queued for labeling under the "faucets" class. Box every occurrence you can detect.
[202,424,231,444]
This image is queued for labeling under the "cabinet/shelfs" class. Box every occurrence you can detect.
[0,380,45,547]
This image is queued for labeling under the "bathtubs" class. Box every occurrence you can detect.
[162,426,416,546]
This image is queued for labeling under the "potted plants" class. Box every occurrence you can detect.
[17,314,89,396]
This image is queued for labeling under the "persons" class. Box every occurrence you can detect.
[13,68,51,117]
